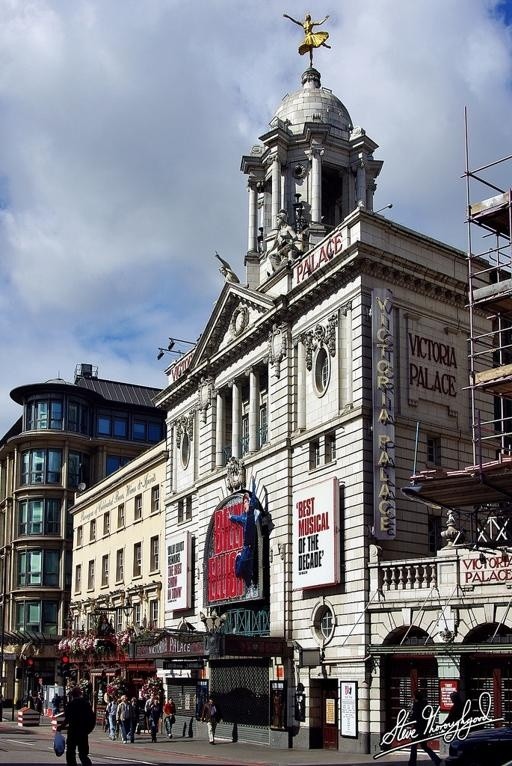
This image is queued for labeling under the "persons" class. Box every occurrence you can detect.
[273,690,282,726]
[226,474,264,598]
[408,690,440,766]
[93,613,116,653]
[215,251,239,283]
[104,690,176,744]
[259,209,298,273]
[52,693,62,716]
[442,692,468,757]
[56,687,95,766]
[200,698,220,745]
[284,13,331,67]
[35,693,44,715]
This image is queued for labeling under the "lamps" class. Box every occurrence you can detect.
[156,336,195,361]
[277,217,335,251]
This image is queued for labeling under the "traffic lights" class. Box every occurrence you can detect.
[62,657,71,677]
[26,660,34,678]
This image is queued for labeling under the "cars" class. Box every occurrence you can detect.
[442,727,511,766]
